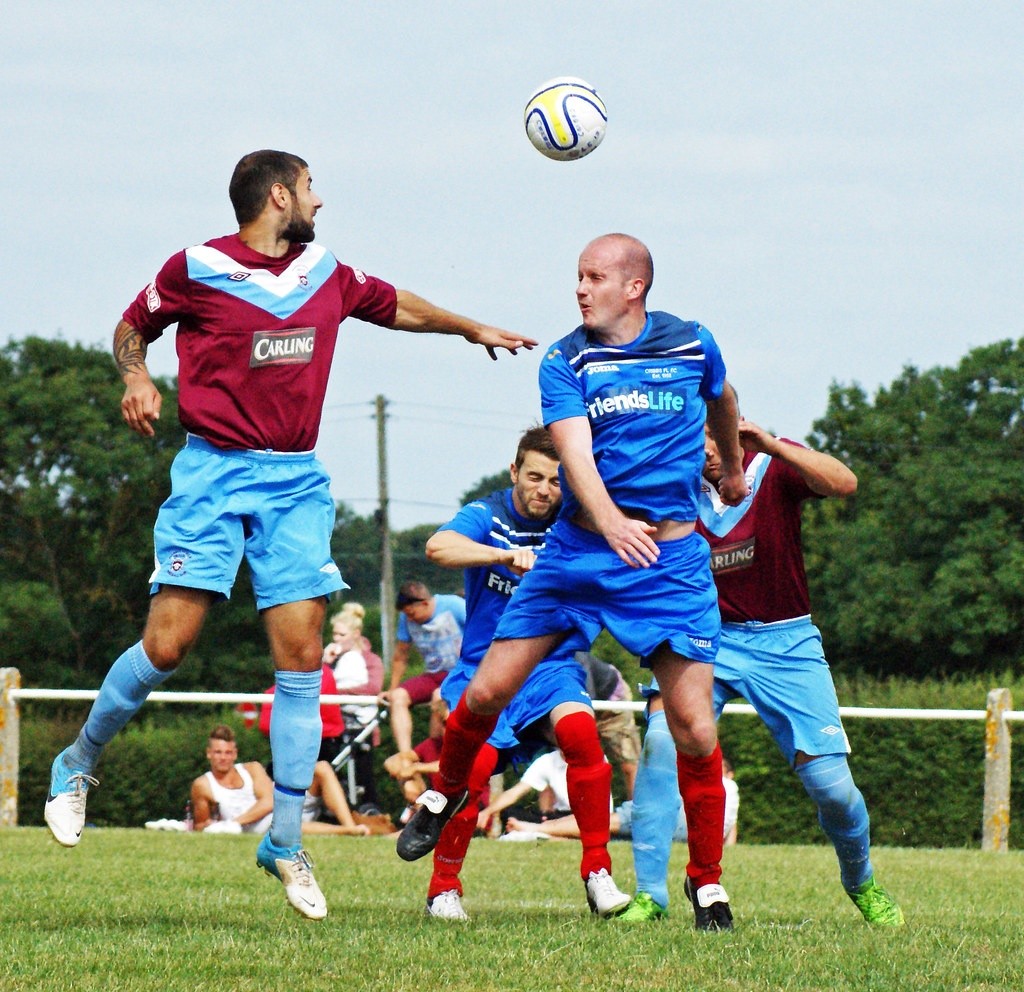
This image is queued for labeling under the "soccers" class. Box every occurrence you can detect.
[523,76,609,162]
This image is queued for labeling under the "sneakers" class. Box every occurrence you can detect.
[425,889,471,922]
[584,867,631,916]
[609,890,667,924]
[256,832,328,920]
[395,785,471,861]
[44,746,100,846]
[682,873,736,932]
[840,869,904,927]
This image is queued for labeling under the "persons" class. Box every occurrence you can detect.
[191,725,370,837]
[397,234,750,933]
[425,426,633,923]
[475,651,739,846]
[44,149,539,919]
[607,378,904,931]
[377,581,509,839]
[321,602,385,814]
